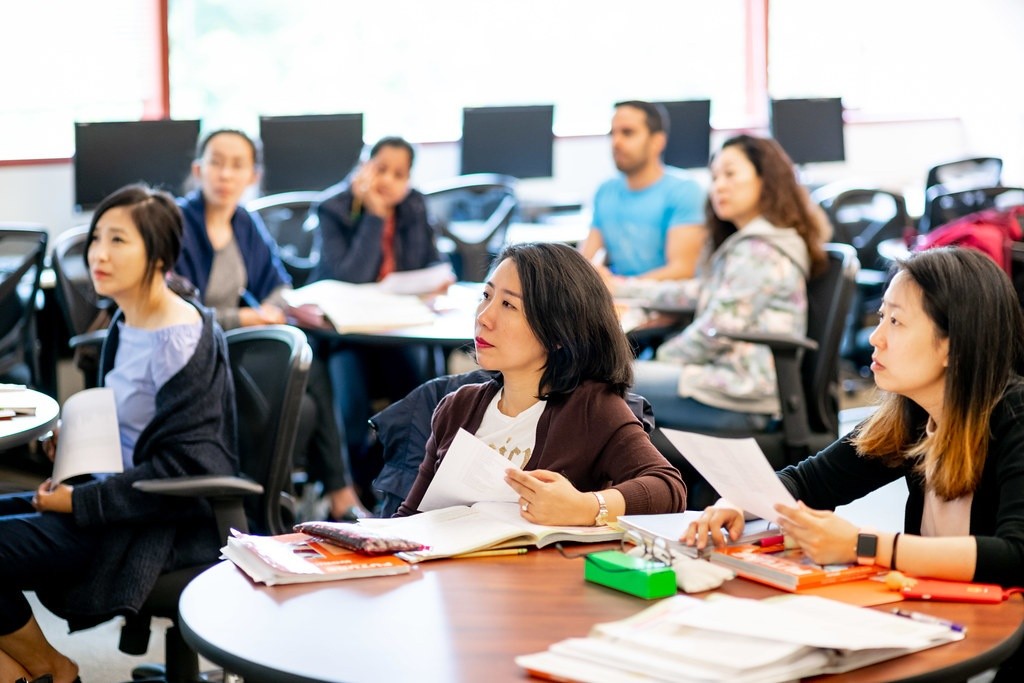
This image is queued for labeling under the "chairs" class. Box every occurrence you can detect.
[51,230,117,346]
[644,241,865,472]
[927,185,1024,265]
[924,156,1004,194]
[423,181,516,286]
[249,195,320,289]
[0,226,48,394]
[125,325,314,683]
[827,190,912,398]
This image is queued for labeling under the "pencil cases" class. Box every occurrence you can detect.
[293,521,428,554]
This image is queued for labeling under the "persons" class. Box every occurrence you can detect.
[0,182,234,683]
[167,129,457,523]
[576,101,834,432]
[391,244,688,526]
[680,246,1024,587]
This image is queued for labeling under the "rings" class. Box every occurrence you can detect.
[522,502,530,511]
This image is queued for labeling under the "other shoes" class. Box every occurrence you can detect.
[327,503,376,523]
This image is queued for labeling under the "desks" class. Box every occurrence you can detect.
[0,380,66,451]
[304,293,692,377]
[174,541,1024,683]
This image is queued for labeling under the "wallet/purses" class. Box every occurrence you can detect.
[293,520,429,555]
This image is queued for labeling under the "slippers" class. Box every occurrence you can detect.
[11,674,82,683]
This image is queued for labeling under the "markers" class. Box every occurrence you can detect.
[751,535,794,547]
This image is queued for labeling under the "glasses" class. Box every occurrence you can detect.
[555,530,675,573]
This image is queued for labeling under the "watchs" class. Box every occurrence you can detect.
[593,492,608,526]
[857,534,878,565]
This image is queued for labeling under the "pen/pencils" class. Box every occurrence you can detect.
[896,605,965,633]
[239,286,258,306]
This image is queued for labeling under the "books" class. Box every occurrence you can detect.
[514,594,964,683]
[353,501,623,563]
[219,527,409,587]
[617,511,888,594]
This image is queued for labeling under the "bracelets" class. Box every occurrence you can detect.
[891,532,901,570]
